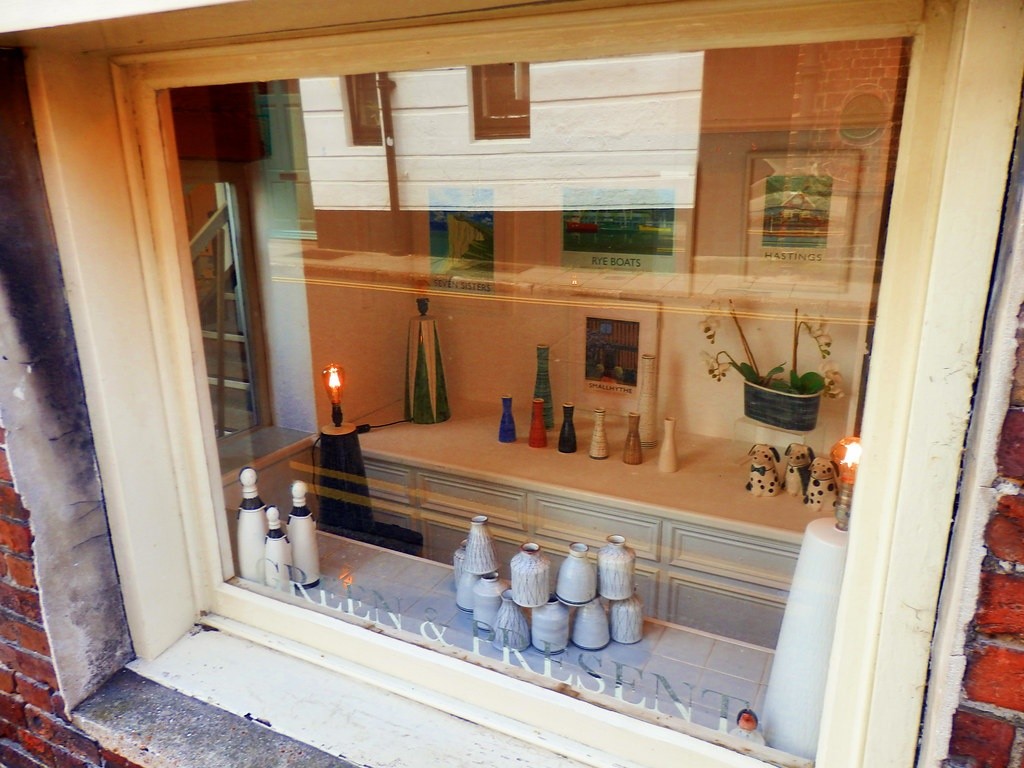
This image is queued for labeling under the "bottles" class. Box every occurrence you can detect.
[235,466,321,594]
[588,407,610,459]
[622,412,643,465]
[528,398,548,448]
[657,417,680,472]
[635,354,659,448]
[452,515,644,654]
[533,342,555,432]
[558,402,577,453]
[498,394,516,443]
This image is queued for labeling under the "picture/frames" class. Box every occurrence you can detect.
[420,212,516,306]
[545,164,701,299]
[741,147,865,294]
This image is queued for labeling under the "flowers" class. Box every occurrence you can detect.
[699,313,843,399]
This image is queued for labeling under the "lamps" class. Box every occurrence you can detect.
[321,363,375,531]
[830,437,861,530]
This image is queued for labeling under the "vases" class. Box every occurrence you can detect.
[500,344,678,473]
[743,382,818,433]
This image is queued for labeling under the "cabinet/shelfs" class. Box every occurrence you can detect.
[362,461,800,648]
